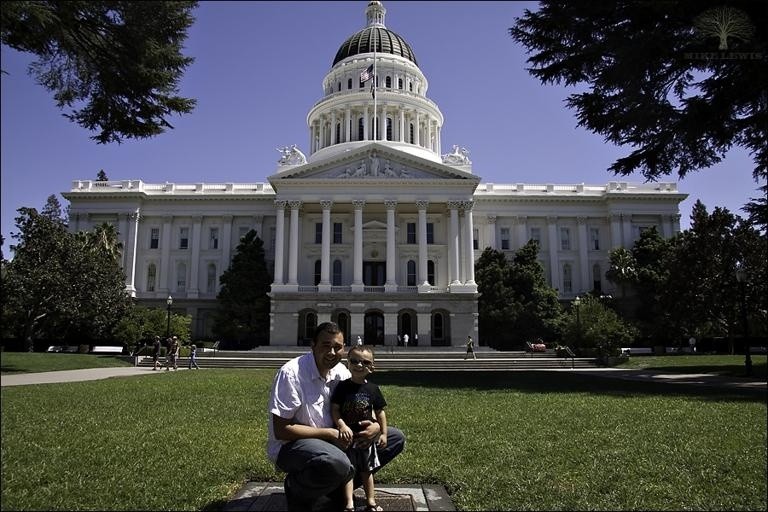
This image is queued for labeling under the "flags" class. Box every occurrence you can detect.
[360,64,374,83]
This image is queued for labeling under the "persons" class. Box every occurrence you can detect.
[464,336,476,360]
[357,335,362,347]
[165,336,180,371]
[331,345,388,512]
[189,344,199,369]
[153,336,163,370]
[403,334,409,348]
[689,336,696,352]
[267,322,406,512]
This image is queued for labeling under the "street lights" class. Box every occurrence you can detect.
[736,268,754,369]
[574,295,582,358]
[166,295,173,339]
[600,294,612,310]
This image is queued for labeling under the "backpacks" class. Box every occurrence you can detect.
[472,341,474,347]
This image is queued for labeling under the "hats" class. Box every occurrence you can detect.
[468,335,471,338]
[173,336,177,339]
[154,336,160,339]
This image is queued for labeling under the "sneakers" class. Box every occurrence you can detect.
[151,365,179,371]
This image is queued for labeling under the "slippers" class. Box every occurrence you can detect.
[343,506,356,512]
[367,503,384,511]
